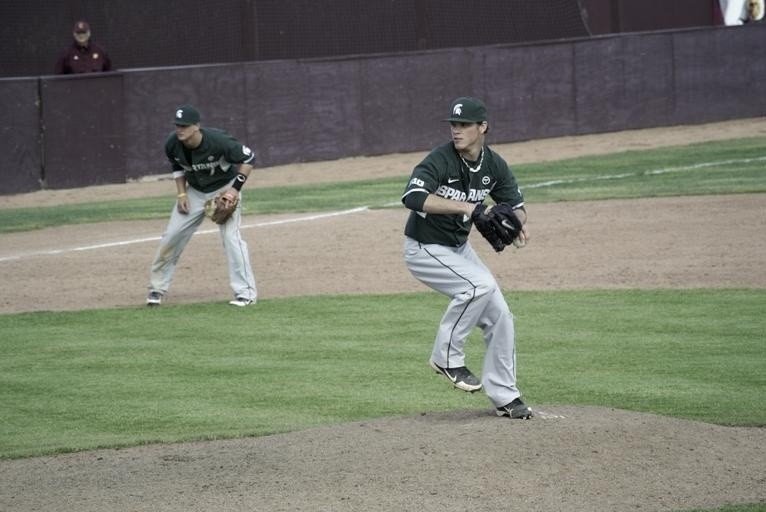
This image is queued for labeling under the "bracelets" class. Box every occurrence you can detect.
[176,193,186,198]
[232,172,248,192]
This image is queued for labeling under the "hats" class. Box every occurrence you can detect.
[74,22,90,33]
[171,106,200,126]
[439,96,487,123]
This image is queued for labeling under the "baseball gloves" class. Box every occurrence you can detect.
[471,202,522,252]
[204,188,239,224]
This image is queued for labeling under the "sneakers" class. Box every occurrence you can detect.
[146,292,162,304]
[429,356,482,392]
[495,397,533,420]
[229,298,252,307]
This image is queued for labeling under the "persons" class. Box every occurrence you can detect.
[143,104,259,308]
[54,20,113,75]
[399,96,535,420]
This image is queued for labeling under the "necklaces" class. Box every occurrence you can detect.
[458,149,484,173]
